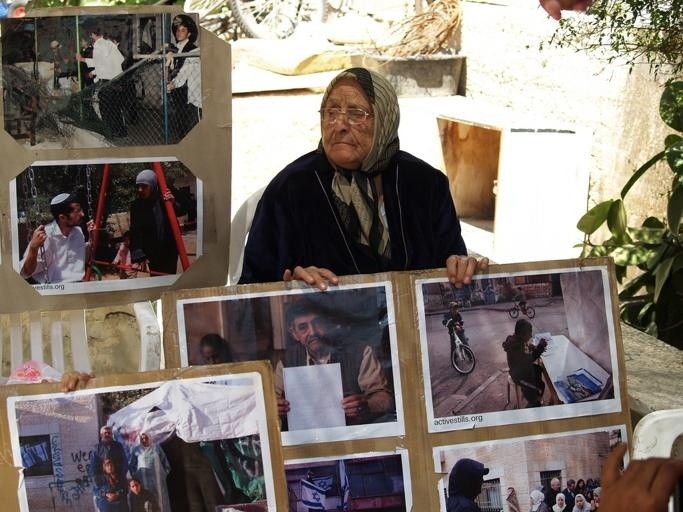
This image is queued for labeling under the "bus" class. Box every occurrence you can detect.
[284,454,404,512]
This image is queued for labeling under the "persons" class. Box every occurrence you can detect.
[19,192,97,285]
[89,425,171,512]
[274,301,394,432]
[199,333,233,365]
[128,169,179,277]
[442,302,473,368]
[59,371,95,393]
[596,442,682,512]
[505,477,602,512]
[237,67,489,291]
[51,14,201,144]
[446,458,489,512]
[502,318,547,408]
[112,231,146,280]
[512,287,527,315]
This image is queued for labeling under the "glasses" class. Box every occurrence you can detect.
[317,107,374,125]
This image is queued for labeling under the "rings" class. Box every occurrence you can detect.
[358,407,363,412]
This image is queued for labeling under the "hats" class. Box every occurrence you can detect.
[483,468,489,475]
[50,192,74,209]
[172,14,198,43]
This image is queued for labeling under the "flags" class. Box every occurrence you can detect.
[300,460,350,512]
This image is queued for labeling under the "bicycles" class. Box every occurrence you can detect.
[509,297,535,319]
[445,318,476,376]
[180,0,335,43]
